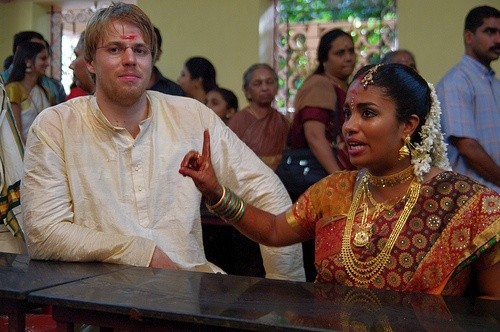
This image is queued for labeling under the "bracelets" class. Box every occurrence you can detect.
[206,185,246,224]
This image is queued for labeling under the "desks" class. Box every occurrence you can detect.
[0,252,500,332]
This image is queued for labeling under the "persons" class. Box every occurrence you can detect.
[1,74,28,253]
[4,42,54,146]
[380,50,418,74]
[227,64,292,173]
[0,31,66,106]
[278,28,356,284]
[205,88,239,125]
[145,27,186,96]
[66,82,91,100]
[178,63,499,299]
[177,56,219,105]
[434,6,499,194]
[68,29,97,95]
[19,1,306,332]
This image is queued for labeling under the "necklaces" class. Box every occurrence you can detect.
[249,105,260,121]
[20,82,44,115]
[341,165,422,284]
